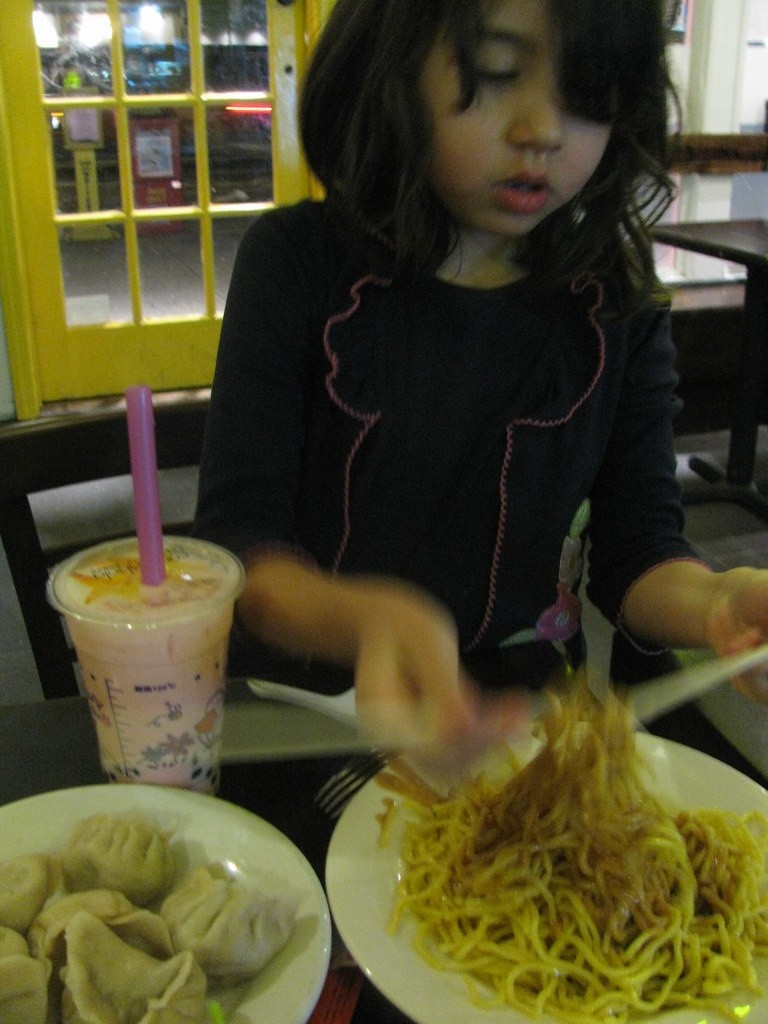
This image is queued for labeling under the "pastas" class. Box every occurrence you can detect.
[376,680,768,1024]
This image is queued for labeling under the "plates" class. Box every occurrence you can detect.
[324,735,768,1024]
[0,783,332,1024]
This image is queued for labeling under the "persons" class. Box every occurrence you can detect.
[191,0,768,763]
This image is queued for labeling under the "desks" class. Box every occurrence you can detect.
[0,634,768,1024]
[641,215,768,519]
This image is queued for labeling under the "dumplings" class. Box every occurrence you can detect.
[0,815,300,1024]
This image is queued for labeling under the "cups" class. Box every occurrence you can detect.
[46,535,247,797]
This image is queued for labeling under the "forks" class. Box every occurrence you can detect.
[313,746,390,817]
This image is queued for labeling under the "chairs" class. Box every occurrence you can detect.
[0,399,209,699]
